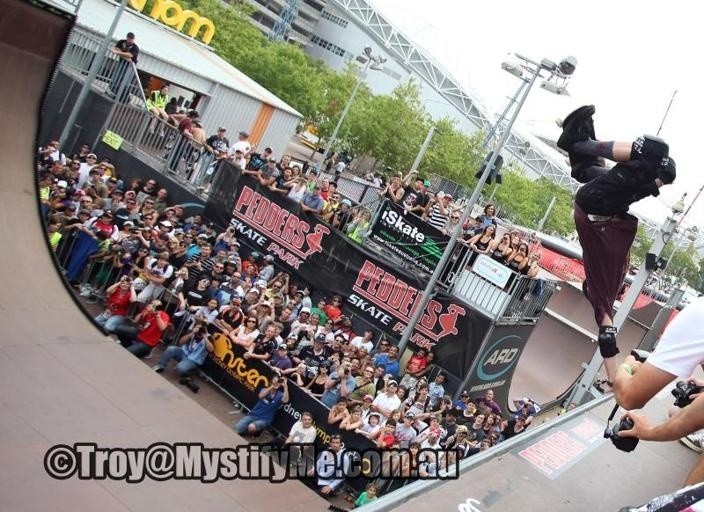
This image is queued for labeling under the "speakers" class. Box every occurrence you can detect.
[475,151,503,184]
[658,257,668,269]
[645,253,656,271]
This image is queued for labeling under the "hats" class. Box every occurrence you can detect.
[250,251,331,366]
[266,146,484,223]
[232,297,242,304]
[66,126,249,259]
[362,379,527,453]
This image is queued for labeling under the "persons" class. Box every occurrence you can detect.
[669,370,704,453]
[555,102,675,391]
[615,395,704,512]
[36,32,553,507]
[615,292,704,410]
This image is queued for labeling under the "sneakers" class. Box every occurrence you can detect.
[557,107,594,151]
[153,364,163,372]
[680,433,704,451]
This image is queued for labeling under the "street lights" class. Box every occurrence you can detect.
[648,224,697,299]
[316,44,388,175]
[536,158,571,233]
[568,191,689,418]
[393,57,577,362]
[475,48,543,157]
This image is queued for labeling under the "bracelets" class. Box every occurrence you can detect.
[621,363,634,376]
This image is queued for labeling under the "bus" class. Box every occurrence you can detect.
[300,121,339,156]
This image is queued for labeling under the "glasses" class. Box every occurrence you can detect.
[331,435,341,438]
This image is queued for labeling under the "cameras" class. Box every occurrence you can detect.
[230,228,234,233]
[278,379,284,383]
[270,360,277,366]
[152,305,162,314]
[179,376,200,393]
[129,227,138,233]
[520,248,524,251]
[523,404,527,408]
[671,381,700,408]
[341,315,349,323]
[503,236,508,239]
[344,368,349,375]
[604,417,639,452]
[125,278,130,282]
[195,327,206,341]
